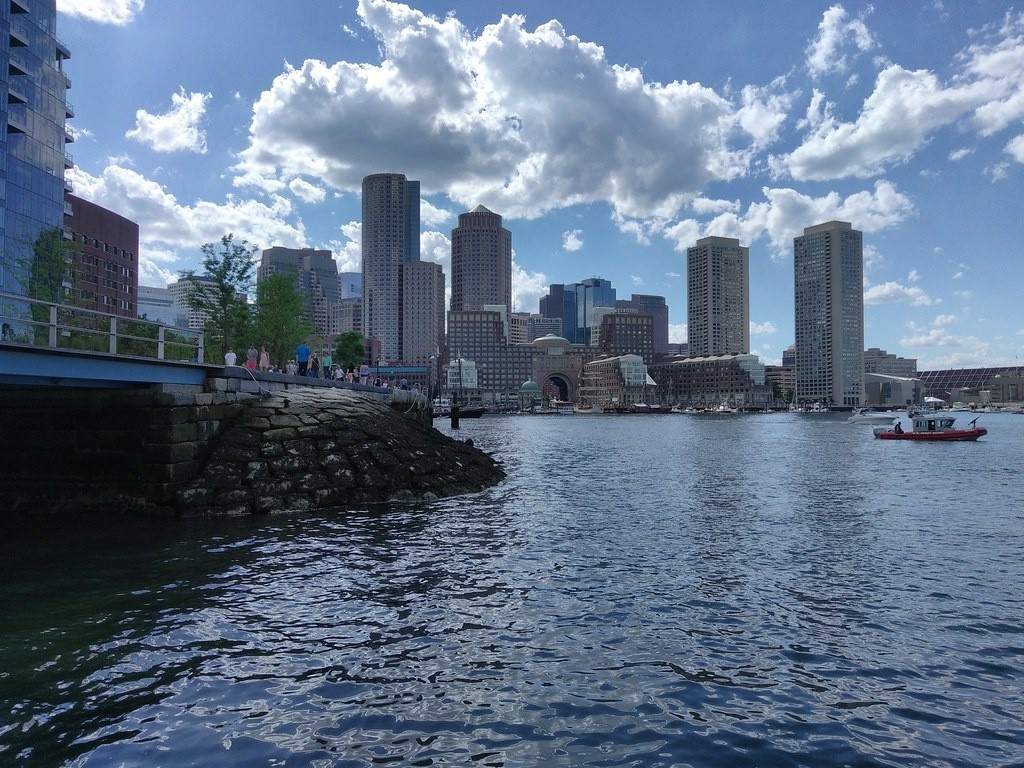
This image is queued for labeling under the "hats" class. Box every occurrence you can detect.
[250,342,254,346]
[287,360,291,363]
[228,347,234,350]
[291,360,295,363]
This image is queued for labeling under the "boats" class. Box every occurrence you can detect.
[872,415,988,441]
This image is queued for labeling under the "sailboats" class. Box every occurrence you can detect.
[448,359,485,418]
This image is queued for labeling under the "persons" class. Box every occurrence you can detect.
[246,342,258,370]
[272,359,297,375]
[259,346,270,371]
[321,351,332,380]
[224,347,237,365]
[311,353,320,378]
[334,361,407,389]
[295,341,312,377]
